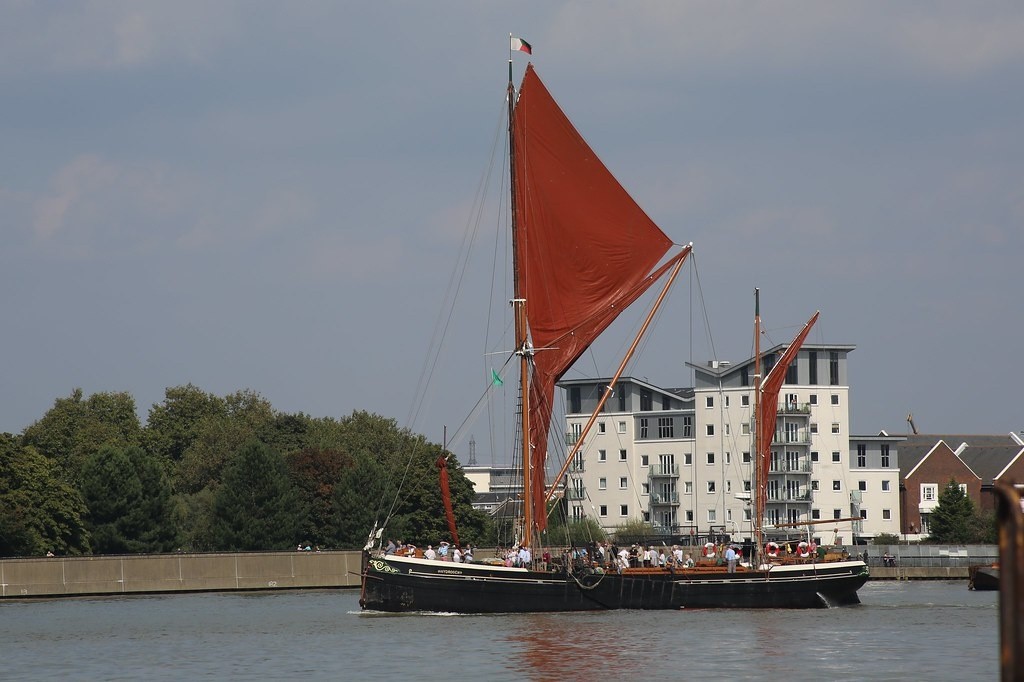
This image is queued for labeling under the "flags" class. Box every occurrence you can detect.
[511,38,531,54]
[492,371,503,386]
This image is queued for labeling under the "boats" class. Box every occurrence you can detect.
[967,562,1001,592]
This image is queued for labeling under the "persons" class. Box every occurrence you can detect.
[297,544,321,553]
[884,552,889,562]
[810,539,817,558]
[864,550,868,562]
[564,541,693,567]
[725,546,736,572]
[505,546,530,568]
[383,539,474,563]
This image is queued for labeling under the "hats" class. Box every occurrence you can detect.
[440,541,446,544]
[631,544,636,547]
[650,546,654,550]
[635,541,639,544]
[407,544,412,546]
[518,546,523,549]
[427,545,432,548]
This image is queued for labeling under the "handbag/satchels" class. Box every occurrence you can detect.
[510,561,513,567]
[505,559,510,566]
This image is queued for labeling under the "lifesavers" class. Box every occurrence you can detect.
[729,545,743,560]
[703,542,717,558]
[797,542,811,558]
[766,542,780,558]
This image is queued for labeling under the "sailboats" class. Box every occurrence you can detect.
[363,29,873,609]
